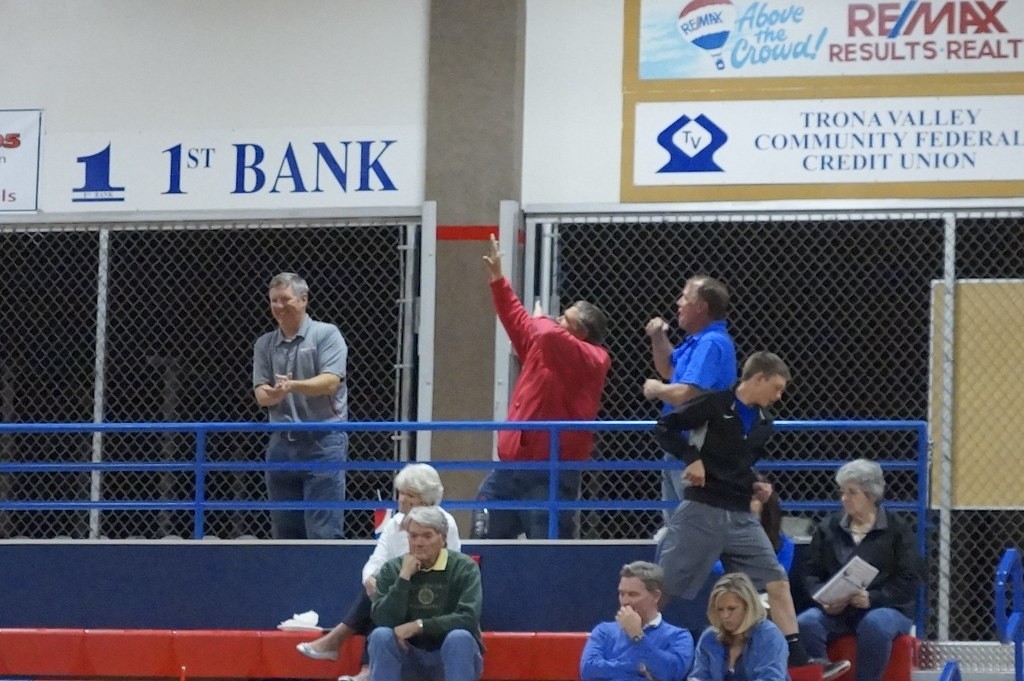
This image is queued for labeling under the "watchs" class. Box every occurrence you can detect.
[630,634,643,643]
[416,619,423,628]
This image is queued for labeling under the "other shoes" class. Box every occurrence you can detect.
[339,675,357,681]
[297,642,339,661]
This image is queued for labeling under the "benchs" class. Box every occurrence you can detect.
[0,628,922,681]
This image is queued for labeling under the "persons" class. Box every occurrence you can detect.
[295,462,462,681]
[580,562,696,681]
[253,271,348,540]
[644,275,736,527]
[790,458,919,681]
[651,352,852,681]
[366,505,487,681]
[469,232,610,540]
[689,572,790,681]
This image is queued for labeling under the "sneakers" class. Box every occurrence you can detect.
[803,657,851,680]
